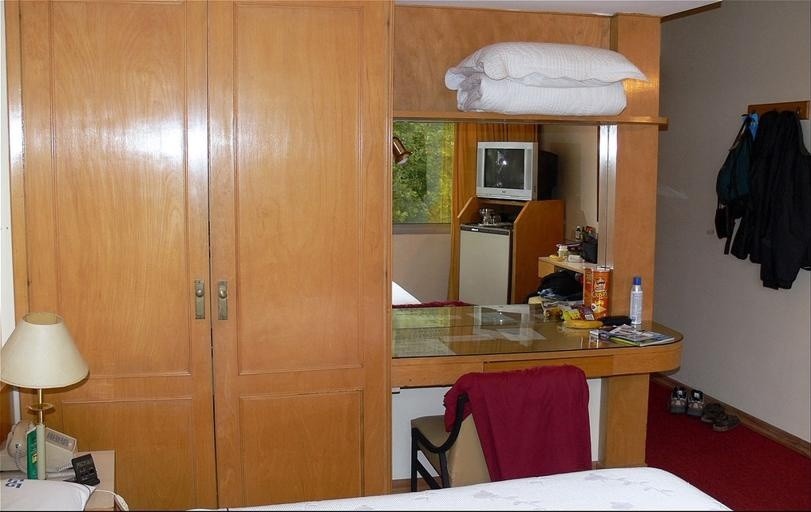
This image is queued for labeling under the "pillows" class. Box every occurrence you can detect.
[444,41,648,91]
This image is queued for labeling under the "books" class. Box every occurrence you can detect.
[590,323,675,347]
[540,299,583,311]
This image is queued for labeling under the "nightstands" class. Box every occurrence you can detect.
[0,450,116,512]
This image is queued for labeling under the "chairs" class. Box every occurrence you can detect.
[411,365,589,494]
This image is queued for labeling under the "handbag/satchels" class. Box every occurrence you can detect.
[715,207,728,239]
[716,129,747,218]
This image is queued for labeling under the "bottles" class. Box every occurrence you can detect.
[583,267,597,306]
[559,245,568,261]
[630,276,644,325]
[575,225,594,242]
[592,267,611,320]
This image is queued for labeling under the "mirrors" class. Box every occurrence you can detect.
[391,120,610,331]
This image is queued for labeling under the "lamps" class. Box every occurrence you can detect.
[0,311,89,481]
[393,136,413,165]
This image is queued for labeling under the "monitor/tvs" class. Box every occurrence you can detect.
[476,141,559,201]
[471,303,545,340]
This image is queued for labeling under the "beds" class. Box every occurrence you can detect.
[196,467,734,512]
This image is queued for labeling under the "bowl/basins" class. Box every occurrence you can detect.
[568,255,582,263]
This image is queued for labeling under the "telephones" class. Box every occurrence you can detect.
[0,420,78,473]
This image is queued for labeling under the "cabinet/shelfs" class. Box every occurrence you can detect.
[4,0,395,512]
[456,195,565,304]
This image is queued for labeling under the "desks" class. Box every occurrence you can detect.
[538,256,597,279]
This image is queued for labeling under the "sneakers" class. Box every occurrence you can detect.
[701,403,724,423]
[713,413,740,431]
[686,389,704,416]
[670,386,687,413]
